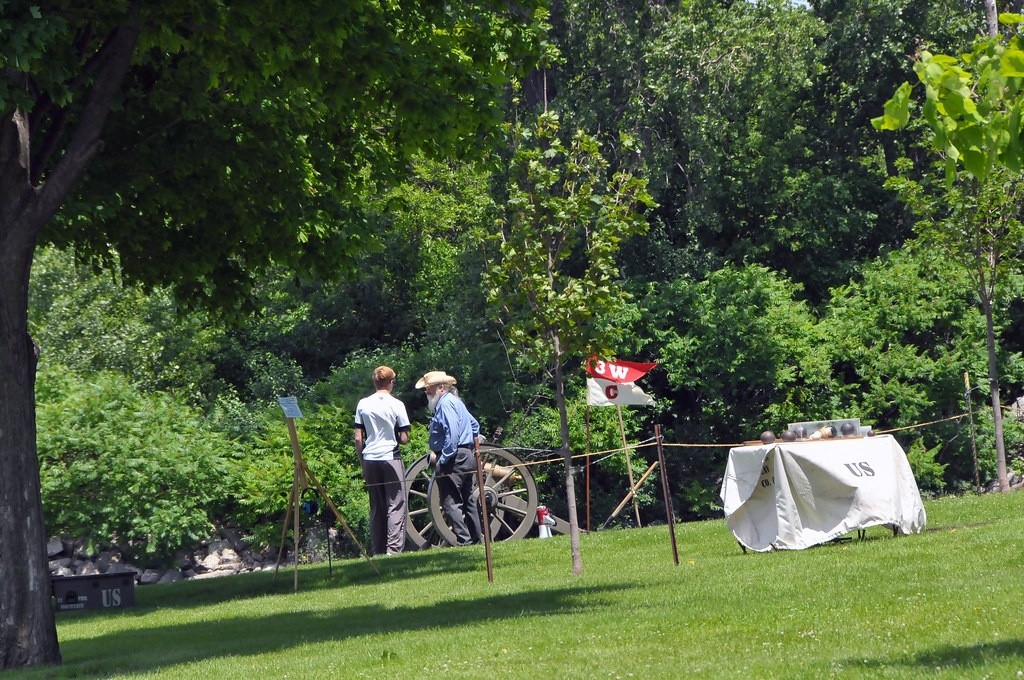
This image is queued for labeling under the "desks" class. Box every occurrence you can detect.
[719,434,927,553]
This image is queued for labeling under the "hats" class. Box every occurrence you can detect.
[415,372,457,390]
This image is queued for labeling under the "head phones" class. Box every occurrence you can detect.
[301,488,320,515]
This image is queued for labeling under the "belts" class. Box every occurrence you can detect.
[435,444,470,455]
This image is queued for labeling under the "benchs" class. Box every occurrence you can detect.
[53,570,138,613]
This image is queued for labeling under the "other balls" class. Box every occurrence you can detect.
[840,423,855,435]
[761,431,775,445]
[797,427,807,438]
[782,430,796,442]
[825,425,838,438]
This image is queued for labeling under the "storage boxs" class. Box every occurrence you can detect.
[787,418,860,437]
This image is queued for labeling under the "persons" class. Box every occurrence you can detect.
[353,366,411,557]
[415,371,485,547]
